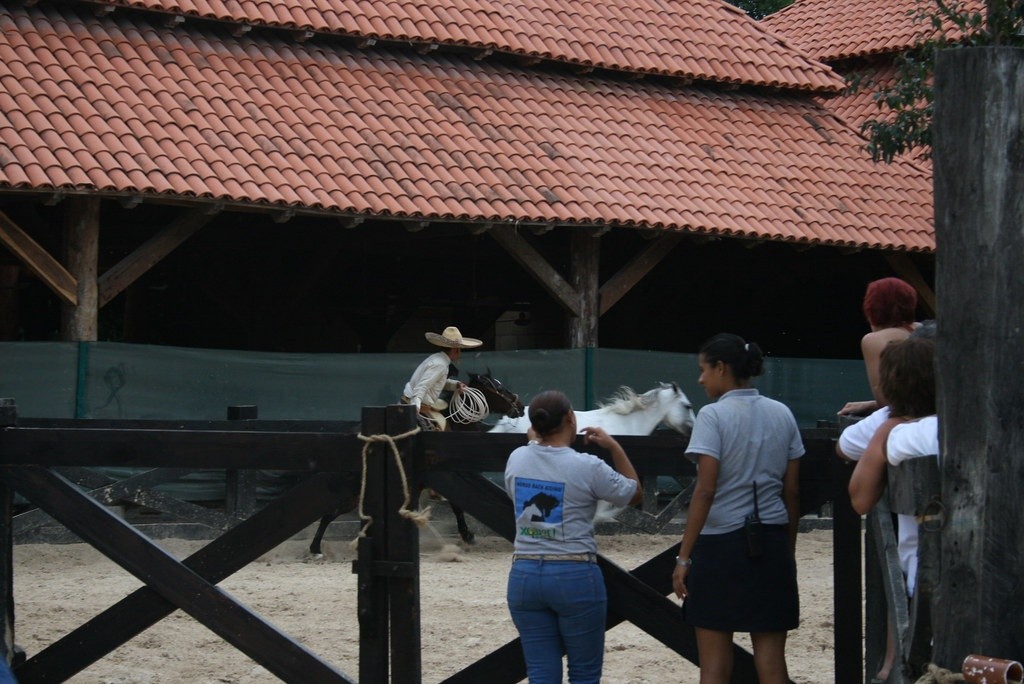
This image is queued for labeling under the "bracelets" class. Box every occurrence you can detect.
[675,556,693,569]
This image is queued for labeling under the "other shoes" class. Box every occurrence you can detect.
[425,488,448,505]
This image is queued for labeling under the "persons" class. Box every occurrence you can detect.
[674,333,806,684]
[504,391,638,684]
[833,278,939,684]
[397,326,484,434]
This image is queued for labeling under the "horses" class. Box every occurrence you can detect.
[304,364,697,563]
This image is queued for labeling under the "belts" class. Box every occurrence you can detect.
[515,553,598,563]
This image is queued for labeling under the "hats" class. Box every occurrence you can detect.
[425,327,483,349]
